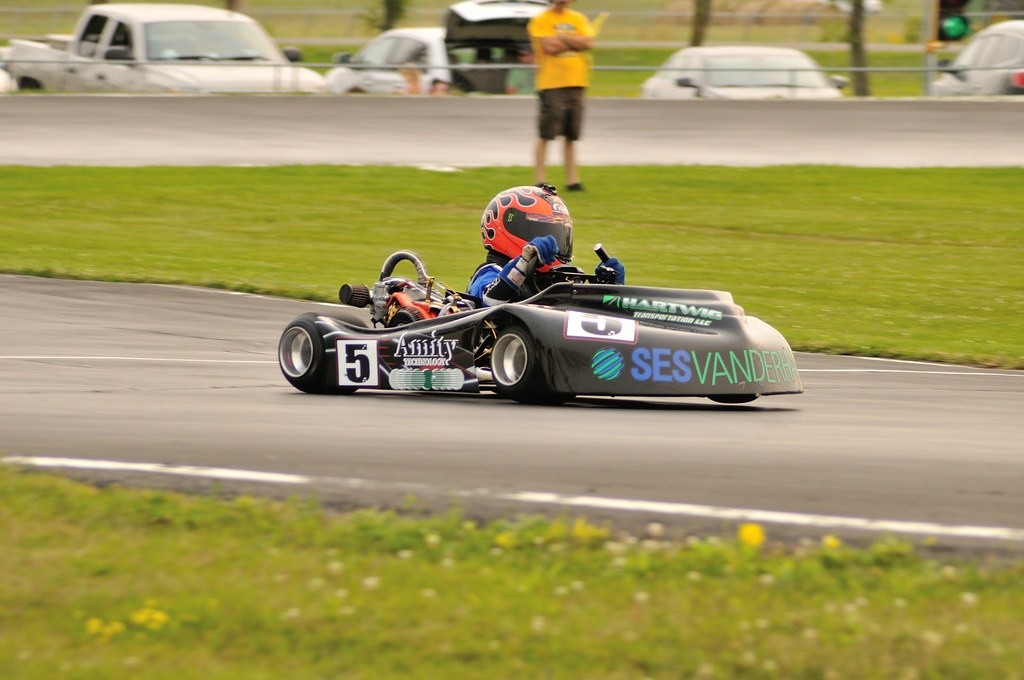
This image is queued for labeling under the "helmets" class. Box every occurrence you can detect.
[480,186,573,273]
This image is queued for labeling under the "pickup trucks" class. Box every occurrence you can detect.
[7,3,331,96]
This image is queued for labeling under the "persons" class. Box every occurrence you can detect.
[505,46,541,96]
[464,186,624,309]
[527,0,596,190]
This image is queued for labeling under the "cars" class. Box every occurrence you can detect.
[325,0,554,94]
[925,21,1024,97]
[639,44,846,104]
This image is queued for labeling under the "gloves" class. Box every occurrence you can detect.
[596,258,625,285]
[496,234,560,292]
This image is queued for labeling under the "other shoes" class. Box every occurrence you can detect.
[567,183,585,192]
